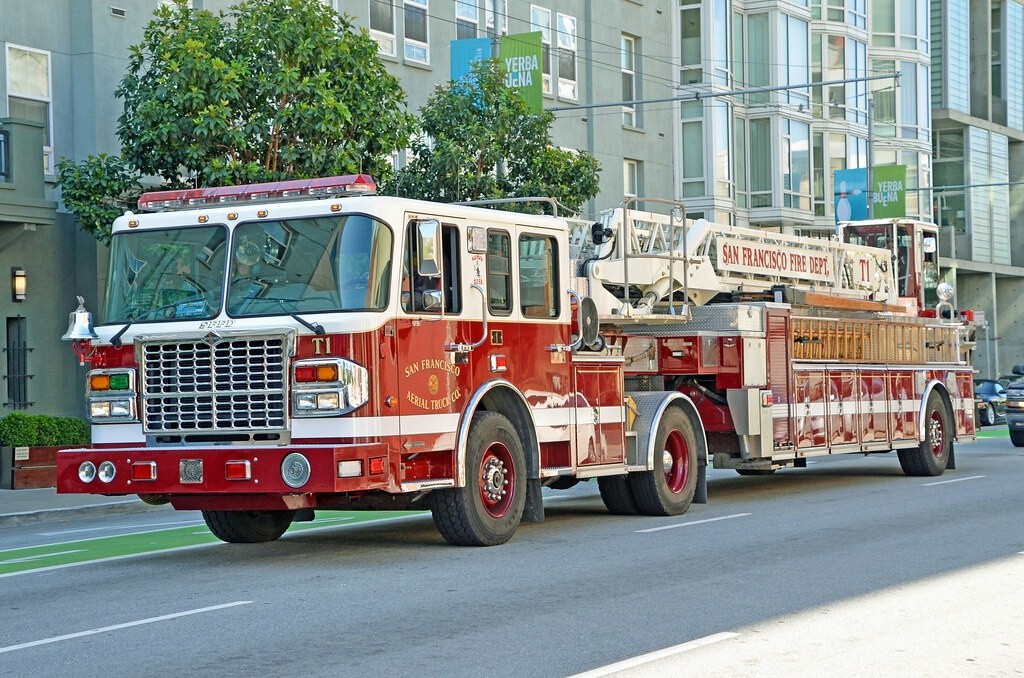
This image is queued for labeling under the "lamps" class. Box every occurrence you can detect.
[11,267,26,302]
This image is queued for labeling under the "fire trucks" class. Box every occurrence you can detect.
[56,172,987,547]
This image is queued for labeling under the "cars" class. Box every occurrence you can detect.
[1006,365,1024,447]
[973,378,1006,426]
